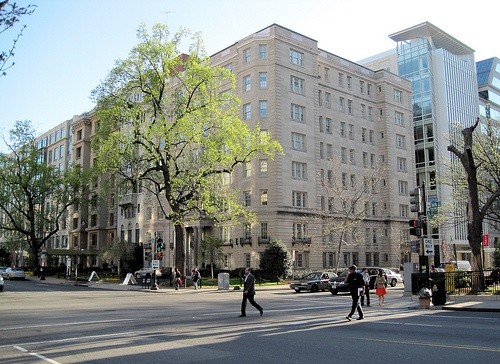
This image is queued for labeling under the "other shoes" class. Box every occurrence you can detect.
[346,316,352,321]
[260,308,263,316]
[356,316,364,320]
[238,314,247,317]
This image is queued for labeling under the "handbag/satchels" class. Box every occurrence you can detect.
[178,279,182,285]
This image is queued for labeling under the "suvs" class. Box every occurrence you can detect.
[327,267,388,295]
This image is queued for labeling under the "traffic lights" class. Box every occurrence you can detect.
[145,251,152,262]
[156,237,164,261]
[409,187,421,213]
[408,219,421,236]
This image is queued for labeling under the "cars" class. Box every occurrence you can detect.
[0,266,26,292]
[289,272,338,293]
[422,268,445,272]
[382,268,403,287]
[134,268,164,279]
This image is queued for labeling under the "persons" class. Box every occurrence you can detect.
[191,267,201,289]
[238,267,263,317]
[359,268,371,306]
[374,268,387,305]
[344,265,365,321]
[174,267,183,290]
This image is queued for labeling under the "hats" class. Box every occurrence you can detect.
[349,265,357,269]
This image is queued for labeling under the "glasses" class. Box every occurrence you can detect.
[349,268,354,270]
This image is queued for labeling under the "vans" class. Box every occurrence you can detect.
[440,261,471,272]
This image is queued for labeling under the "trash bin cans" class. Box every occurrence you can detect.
[142,273,151,286]
[412,272,429,293]
[430,272,446,304]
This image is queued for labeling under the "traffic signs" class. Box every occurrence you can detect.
[423,238,435,256]
[152,260,160,271]
